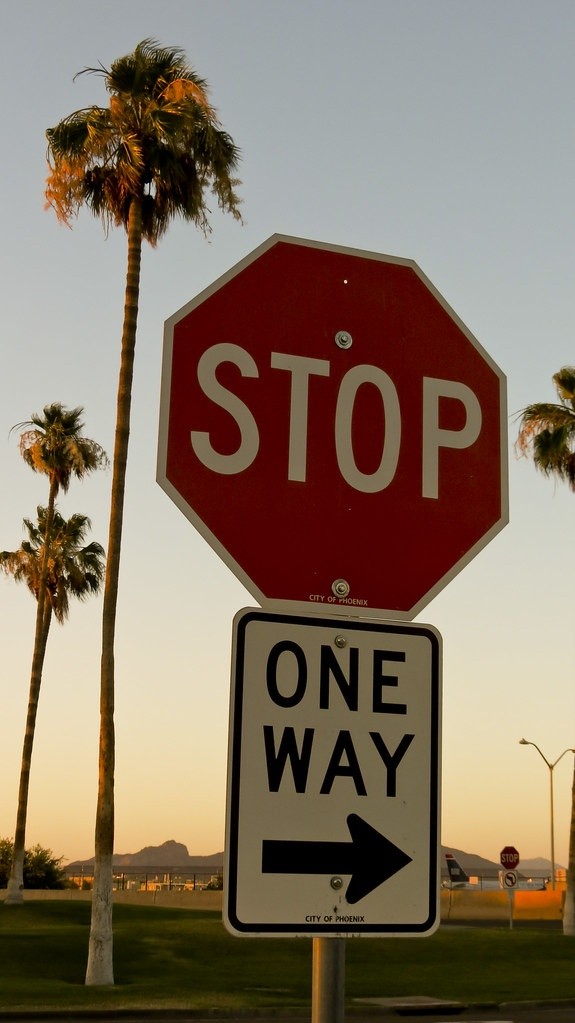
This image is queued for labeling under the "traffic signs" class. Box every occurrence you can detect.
[222,607,444,941]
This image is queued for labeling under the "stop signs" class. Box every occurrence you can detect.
[157,231,509,622]
[500,846,520,871]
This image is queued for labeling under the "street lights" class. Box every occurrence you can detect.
[518,737,575,889]
